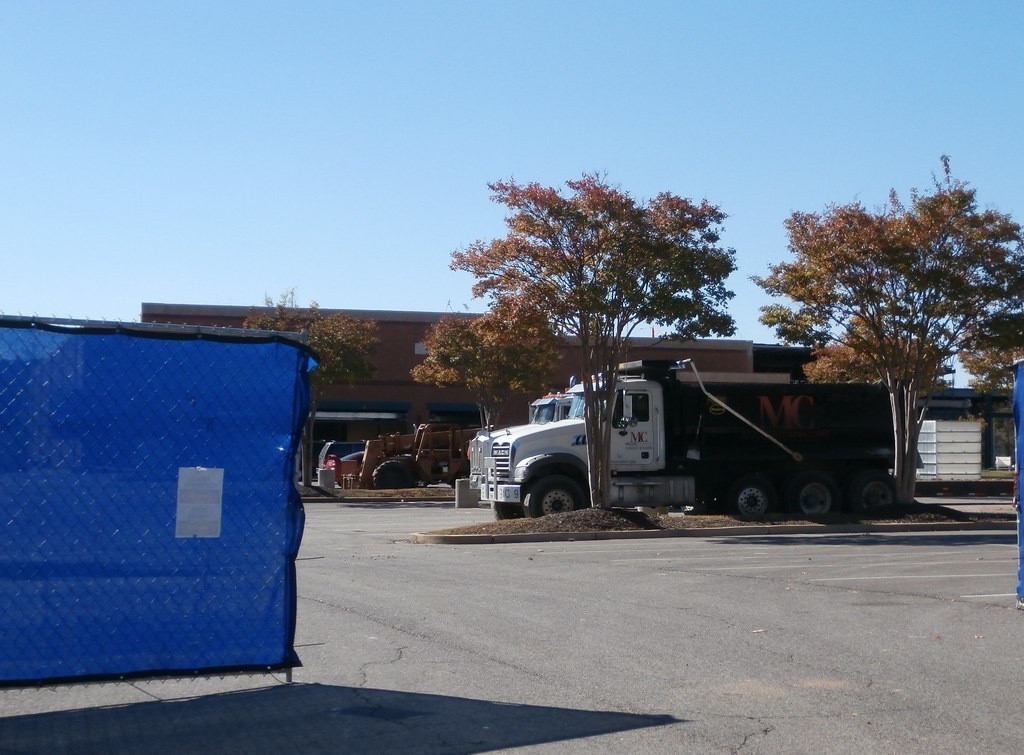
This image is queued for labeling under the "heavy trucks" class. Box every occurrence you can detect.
[467,359,926,522]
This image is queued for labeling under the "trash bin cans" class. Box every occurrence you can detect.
[318,467,336,488]
[455,477,479,507]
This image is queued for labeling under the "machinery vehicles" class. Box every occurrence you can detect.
[331,423,486,490]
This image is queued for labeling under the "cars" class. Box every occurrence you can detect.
[313,436,366,487]
